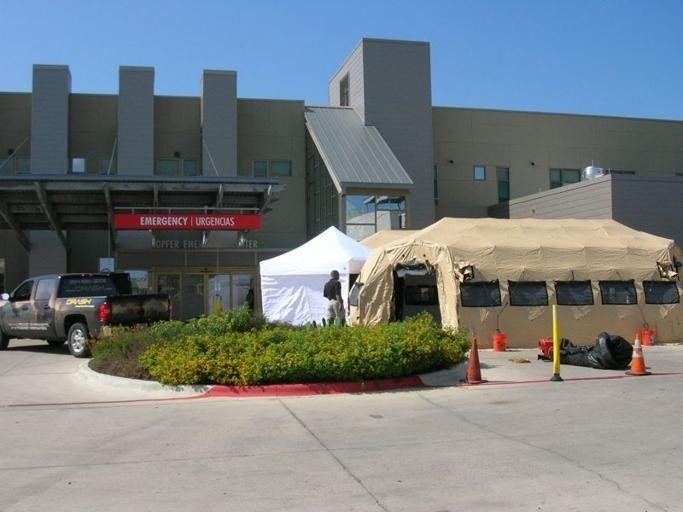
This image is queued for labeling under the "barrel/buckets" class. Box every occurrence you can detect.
[494,334,507,351]
[642,329,656,346]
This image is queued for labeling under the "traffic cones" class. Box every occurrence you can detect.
[459,337,488,385]
[623,331,653,378]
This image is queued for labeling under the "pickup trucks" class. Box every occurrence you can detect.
[0,270,172,361]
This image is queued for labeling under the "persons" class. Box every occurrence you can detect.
[323,270,348,326]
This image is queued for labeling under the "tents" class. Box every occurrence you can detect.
[346,215,681,350]
[257,225,372,327]
